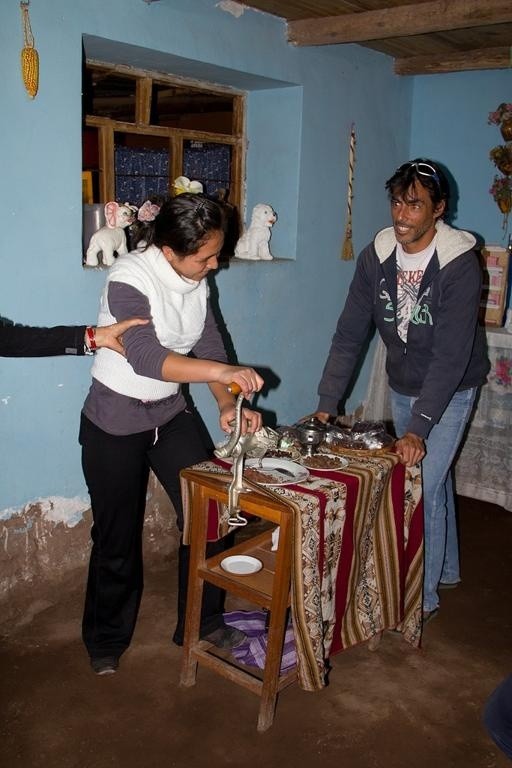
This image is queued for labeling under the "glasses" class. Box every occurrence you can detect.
[396,161,441,196]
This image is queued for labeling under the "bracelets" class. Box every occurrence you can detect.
[85,324,97,355]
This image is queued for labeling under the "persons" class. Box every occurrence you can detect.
[78,192,265,677]
[298,157,492,625]
[0,316,150,359]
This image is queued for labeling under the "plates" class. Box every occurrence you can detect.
[275,448,301,461]
[229,457,310,487]
[299,453,349,471]
[220,554,263,574]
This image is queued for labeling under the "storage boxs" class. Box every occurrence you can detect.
[480,242,509,331]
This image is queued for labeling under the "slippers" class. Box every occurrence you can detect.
[386,611,438,636]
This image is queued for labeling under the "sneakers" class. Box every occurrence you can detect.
[91,655,119,675]
[176,624,247,649]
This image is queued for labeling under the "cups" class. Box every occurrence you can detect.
[84,202,108,264]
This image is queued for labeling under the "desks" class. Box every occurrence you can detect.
[357,325,512,515]
[174,446,427,736]
